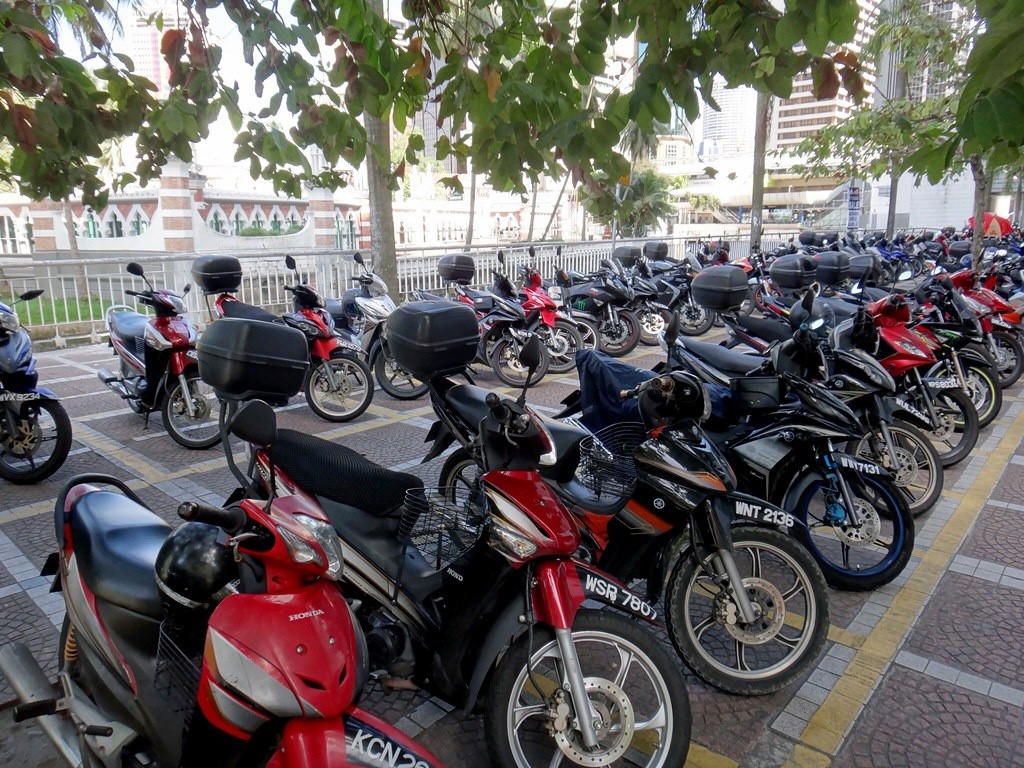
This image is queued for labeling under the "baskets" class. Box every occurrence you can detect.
[154,617,201,730]
[396,486,488,565]
[579,422,650,498]
[729,377,779,416]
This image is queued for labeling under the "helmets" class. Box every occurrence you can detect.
[789,298,835,339]
[153,521,239,632]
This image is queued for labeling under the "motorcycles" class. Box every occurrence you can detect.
[0,221,1024,768]
[0,289,72,485]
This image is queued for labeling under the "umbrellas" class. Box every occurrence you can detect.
[969,212,1014,239]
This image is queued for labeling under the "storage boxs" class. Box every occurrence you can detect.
[194,217,970,397]
